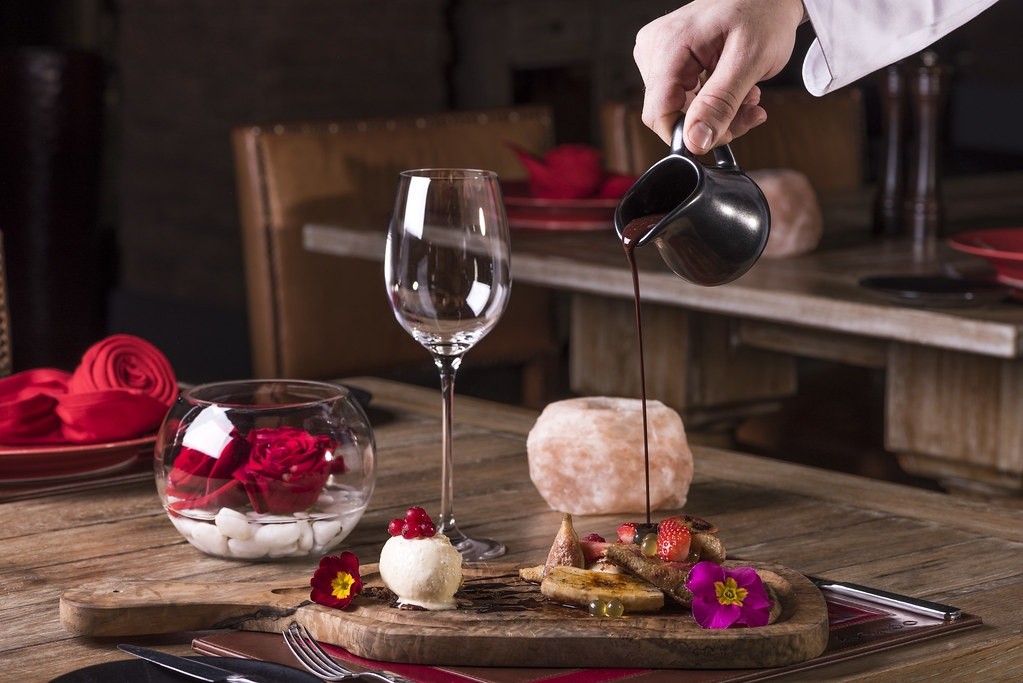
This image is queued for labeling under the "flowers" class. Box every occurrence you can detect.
[685,560,775,630]
[163,424,347,520]
[311,550,365,608]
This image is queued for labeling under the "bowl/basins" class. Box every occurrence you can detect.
[948,227,1023,289]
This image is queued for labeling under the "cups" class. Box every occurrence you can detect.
[602,173,635,198]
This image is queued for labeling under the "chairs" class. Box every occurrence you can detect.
[229,105,561,404]
[599,89,867,193]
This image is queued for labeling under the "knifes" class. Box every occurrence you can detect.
[803,574,962,621]
[117,644,273,683]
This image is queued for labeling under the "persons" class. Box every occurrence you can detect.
[631,0,997,155]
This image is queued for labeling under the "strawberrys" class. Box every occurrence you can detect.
[581,518,691,561]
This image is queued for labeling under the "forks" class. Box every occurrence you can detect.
[282,624,417,683]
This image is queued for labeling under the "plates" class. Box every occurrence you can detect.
[496,179,620,230]
[48,656,324,683]
[864,275,1006,309]
[55,559,829,666]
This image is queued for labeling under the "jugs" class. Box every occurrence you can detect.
[614,114,770,287]
[501,140,604,198]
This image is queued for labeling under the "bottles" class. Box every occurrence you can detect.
[871,51,942,232]
[153,379,377,563]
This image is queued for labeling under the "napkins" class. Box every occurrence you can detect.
[0,334,178,453]
[506,140,624,202]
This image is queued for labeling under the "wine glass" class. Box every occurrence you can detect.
[385,167,513,561]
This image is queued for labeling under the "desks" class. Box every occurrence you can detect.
[0,376,1023,683]
[283,176,1018,498]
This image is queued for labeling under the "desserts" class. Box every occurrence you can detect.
[378,507,461,611]
